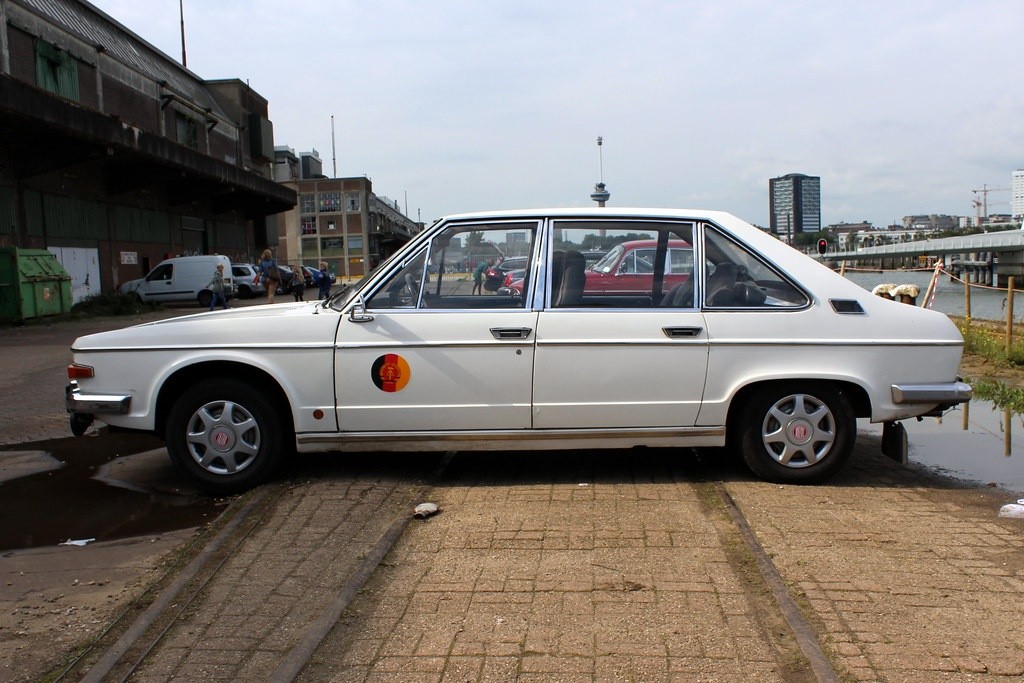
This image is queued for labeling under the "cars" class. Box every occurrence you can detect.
[497,238,714,296]
[503,252,654,286]
[308,268,336,286]
[63,207,971,491]
[276,266,296,295]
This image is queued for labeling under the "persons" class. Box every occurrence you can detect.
[292,263,305,302]
[255,249,281,304]
[206,264,231,311]
[311,262,331,300]
[471,258,494,295]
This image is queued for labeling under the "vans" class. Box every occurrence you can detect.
[120,254,235,307]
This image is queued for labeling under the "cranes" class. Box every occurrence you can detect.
[973,196,981,227]
[972,183,1012,220]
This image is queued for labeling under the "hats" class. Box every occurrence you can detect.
[320,262,327,268]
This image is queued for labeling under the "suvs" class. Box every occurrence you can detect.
[484,255,529,292]
[286,265,315,289]
[230,263,266,299]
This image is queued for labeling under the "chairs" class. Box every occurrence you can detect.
[552,250,586,306]
[659,262,738,306]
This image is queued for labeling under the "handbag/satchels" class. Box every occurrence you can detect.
[268,268,280,281]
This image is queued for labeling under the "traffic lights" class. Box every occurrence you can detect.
[818,238,826,254]
